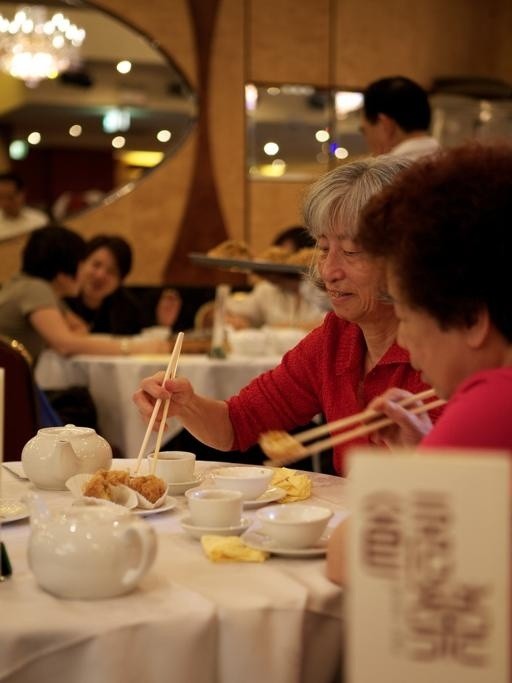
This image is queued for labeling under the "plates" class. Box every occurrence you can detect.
[255,539,330,557]
[167,480,204,495]
[131,496,178,516]
[242,487,288,509]
[177,515,254,541]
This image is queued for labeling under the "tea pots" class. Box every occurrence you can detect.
[21,424,113,491]
[19,490,157,599]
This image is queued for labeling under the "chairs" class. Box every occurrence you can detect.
[195,291,246,331]
[0,332,46,461]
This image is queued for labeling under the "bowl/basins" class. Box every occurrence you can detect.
[227,327,309,358]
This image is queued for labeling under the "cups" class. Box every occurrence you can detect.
[255,503,334,549]
[209,467,273,500]
[147,451,196,482]
[188,489,242,528]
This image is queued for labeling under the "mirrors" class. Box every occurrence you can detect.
[1,0,199,245]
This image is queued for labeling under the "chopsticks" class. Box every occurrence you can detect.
[133,332,185,475]
[264,388,448,467]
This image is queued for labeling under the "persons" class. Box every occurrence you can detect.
[321,139,512,592]
[131,158,452,479]
[67,235,183,333]
[225,225,327,333]
[0,175,51,240]
[0,222,173,458]
[358,71,440,164]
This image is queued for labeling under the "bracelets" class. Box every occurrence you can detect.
[119,333,131,354]
